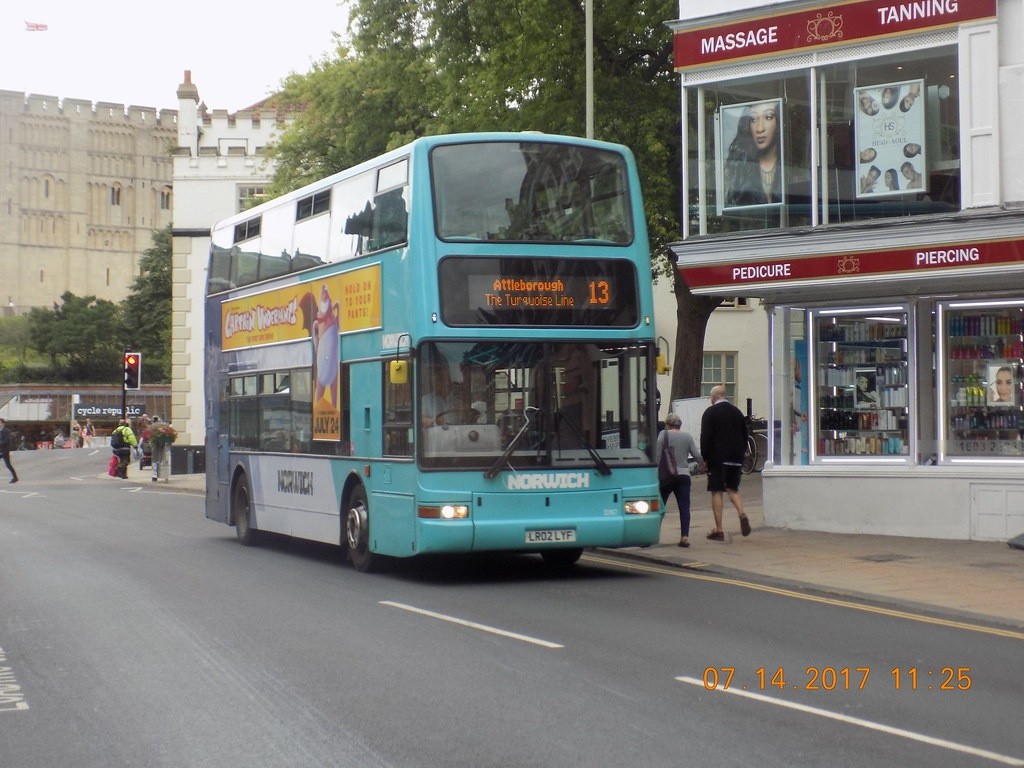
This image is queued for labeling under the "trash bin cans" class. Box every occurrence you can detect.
[169,445,193,475]
[194,445,205,473]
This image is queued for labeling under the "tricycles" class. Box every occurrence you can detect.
[137,428,153,470]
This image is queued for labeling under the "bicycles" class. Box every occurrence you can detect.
[742,416,768,474]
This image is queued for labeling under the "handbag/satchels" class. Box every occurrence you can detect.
[108,454,121,477]
[658,430,678,482]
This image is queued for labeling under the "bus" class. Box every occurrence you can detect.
[204,132,666,570]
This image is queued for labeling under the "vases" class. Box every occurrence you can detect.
[147,440,167,465]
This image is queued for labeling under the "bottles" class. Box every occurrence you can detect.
[946,312,1024,441]
[818,319,911,457]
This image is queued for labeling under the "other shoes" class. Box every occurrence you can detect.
[740,513,751,537]
[9,478,18,484]
[678,536,692,547]
[706,532,724,541]
[152,477,158,481]
[122,475,128,479]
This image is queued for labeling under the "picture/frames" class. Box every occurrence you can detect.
[719,98,785,212]
[853,77,927,200]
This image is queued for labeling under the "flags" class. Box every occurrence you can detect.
[27,21,47,31]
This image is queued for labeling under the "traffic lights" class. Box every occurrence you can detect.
[123,354,139,388]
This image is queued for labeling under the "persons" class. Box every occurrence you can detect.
[723,101,782,206]
[856,375,877,407]
[420,370,464,426]
[987,367,1011,403]
[110,418,138,478]
[654,412,706,548]
[149,416,165,484]
[19,418,96,451]
[138,420,151,447]
[858,83,922,194]
[699,385,750,541]
[0,419,17,483]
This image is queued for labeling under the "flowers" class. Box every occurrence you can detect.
[143,419,178,445]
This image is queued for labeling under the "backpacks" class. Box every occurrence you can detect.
[111,425,127,448]
